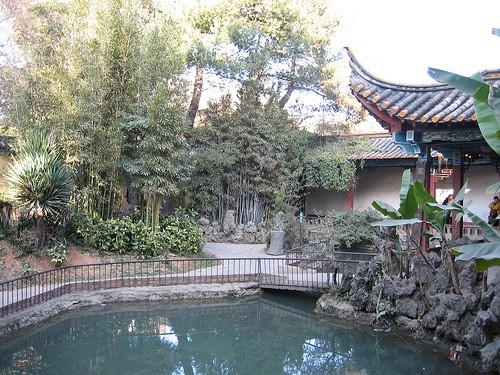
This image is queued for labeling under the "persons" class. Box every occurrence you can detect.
[488,196,500,227]
[442,193,453,223]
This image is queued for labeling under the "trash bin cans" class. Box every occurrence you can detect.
[267,230,286,255]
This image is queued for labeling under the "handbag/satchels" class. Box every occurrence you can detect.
[490,208,498,218]
